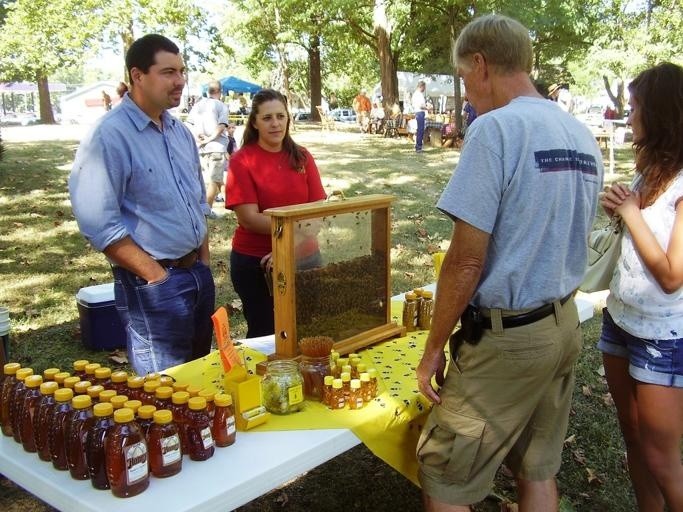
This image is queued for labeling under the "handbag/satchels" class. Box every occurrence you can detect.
[575,227,624,294]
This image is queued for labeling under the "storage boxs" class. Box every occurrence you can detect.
[75,283,127,350]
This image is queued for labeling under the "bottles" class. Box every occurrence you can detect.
[323,348,378,411]
[299,353,331,403]
[402,292,418,332]
[0,359,237,498]
[419,291,435,330]
[413,288,424,327]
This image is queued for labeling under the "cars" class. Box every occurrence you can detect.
[337,109,356,120]
[292,112,311,120]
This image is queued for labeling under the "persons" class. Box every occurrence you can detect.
[416,15,603,511]
[328,92,344,121]
[353,80,476,153]
[102,91,110,111]
[604,104,614,119]
[187,80,248,217]
[111,83,127,107]
[594,62,682,512]
[225,89,327,337]
[70,35,215,375]
[546,83,574,114]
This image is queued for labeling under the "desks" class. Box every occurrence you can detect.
[0,280,610,512]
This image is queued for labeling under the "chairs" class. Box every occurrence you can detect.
[316,105,336,131]
[384,114,402,138]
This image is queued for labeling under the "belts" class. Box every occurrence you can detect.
[475,290,578,333]
[122,248,202,274]
